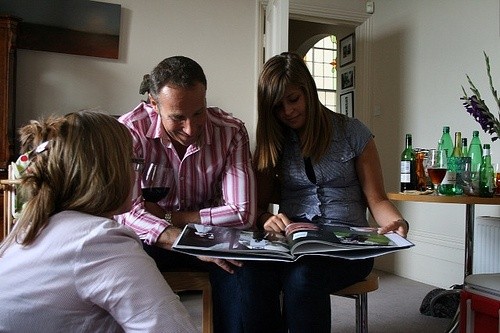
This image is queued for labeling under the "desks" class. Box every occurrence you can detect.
[387,191,500,333]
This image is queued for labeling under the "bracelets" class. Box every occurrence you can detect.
[165,207,172,224]
[398,219,409,234]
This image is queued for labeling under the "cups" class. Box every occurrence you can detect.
[492,162,500,192]
[416,148,430,192]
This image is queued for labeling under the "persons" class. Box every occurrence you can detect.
[0,112,198,333]
[113,56,257,333]
[139,74,156,104]
[246,52,409,333]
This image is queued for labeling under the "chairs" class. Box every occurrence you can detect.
[331,271,379,333]
[459,271,500,333]
[160,271,215,333]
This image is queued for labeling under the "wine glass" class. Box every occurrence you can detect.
[427,148,448,196]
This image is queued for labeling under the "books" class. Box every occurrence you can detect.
[171,224,415,262]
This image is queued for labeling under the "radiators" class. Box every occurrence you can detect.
[473,214,500,274]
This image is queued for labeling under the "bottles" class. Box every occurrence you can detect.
[400,126,500,196]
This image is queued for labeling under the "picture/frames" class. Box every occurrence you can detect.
[339,66,356,93]
[340,90,354,118]
[338,33,356,68]
